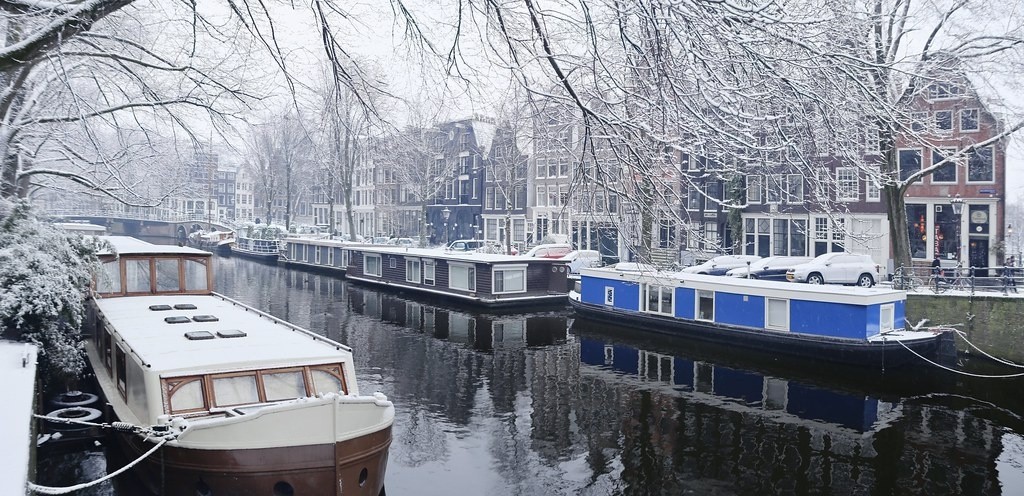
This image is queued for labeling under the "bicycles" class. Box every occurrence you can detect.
[891,270,924,293]
[929,261,975,294]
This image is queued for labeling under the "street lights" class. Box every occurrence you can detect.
[950,193,967,285]
[441,207,452,243]
[1008,223,1012,259]
[267,199,271,226]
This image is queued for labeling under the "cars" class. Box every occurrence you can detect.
[524,244,572,259]
[726,257,815,281]
[289,225,297,233]
[386,238,415,248]
[558,250,602,274]
[365,237,390,245]
[680,255,762,276]
[474,244,518,255]
[786,252,879,288]
[334,234,366,243]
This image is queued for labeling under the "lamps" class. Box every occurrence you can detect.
[1005,224,1012,238]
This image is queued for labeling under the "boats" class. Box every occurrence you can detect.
[568,263,943,363]
[78,236,395,496]
[231,236,283,259]
[188,231,235,250]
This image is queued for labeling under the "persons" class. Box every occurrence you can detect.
[932,255,941,283]
[1001,258,1011,291]
[1010,255,1019,293]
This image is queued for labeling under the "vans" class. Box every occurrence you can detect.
[449,240,496,252]
